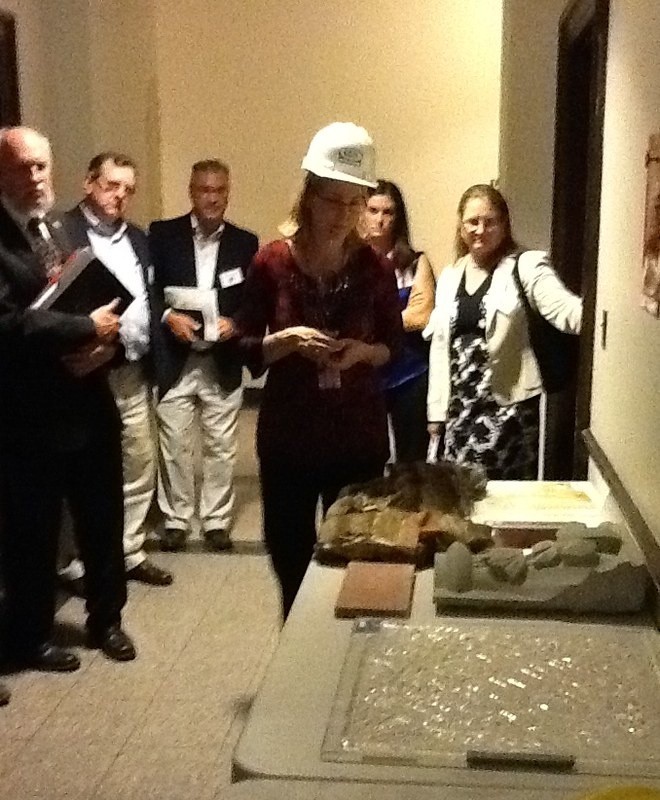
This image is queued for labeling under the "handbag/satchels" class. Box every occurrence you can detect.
[512,250,581,394]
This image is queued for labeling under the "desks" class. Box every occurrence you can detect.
[217,482,660,800]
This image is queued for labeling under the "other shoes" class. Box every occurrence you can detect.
[0,682,11,705]
[159,528,185,552]
[126,560,172,585]
[63,576,88,600]
[86,621,135,660]
[21,639,81,672]
[205,530,233,551]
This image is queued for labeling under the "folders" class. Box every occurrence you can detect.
[31,247,135,316]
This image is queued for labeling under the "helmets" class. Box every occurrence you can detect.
[301,122,378,190]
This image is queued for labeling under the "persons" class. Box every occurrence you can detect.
[143,159,263,553]
[422,185,583,481]
[237,120,407,637]
[62,151,174,599]
[0,125,136,708]
[347,179,437,483]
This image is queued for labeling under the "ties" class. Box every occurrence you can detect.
[26,216,54,276]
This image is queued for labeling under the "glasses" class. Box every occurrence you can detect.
[310,186,367,214]
